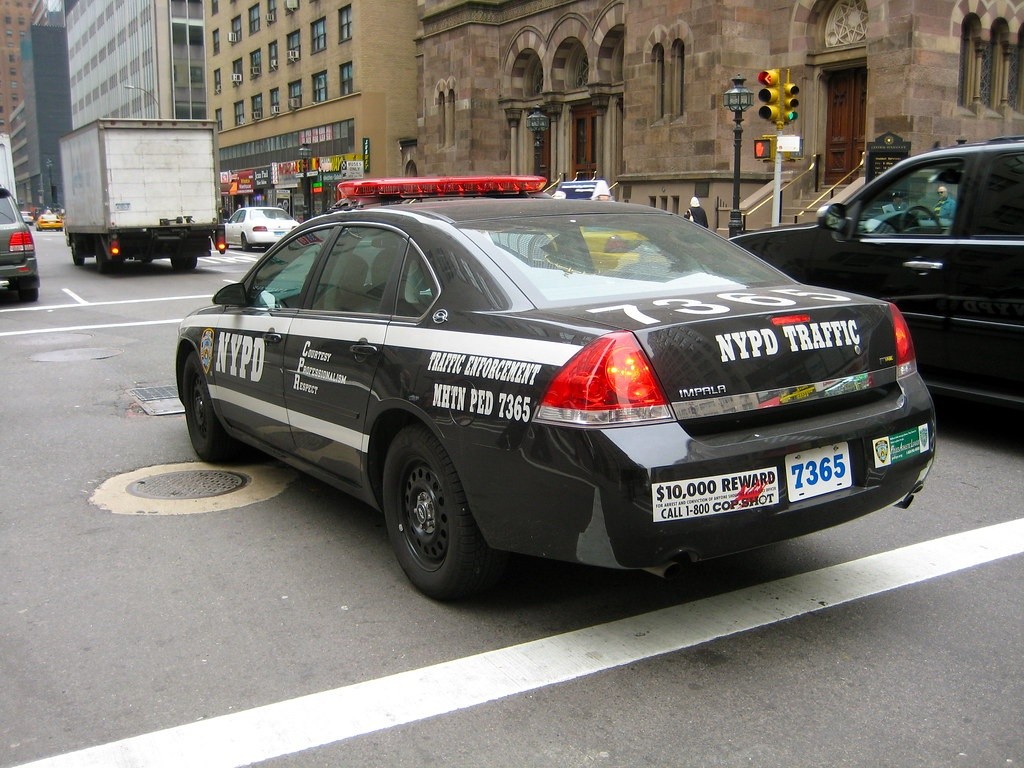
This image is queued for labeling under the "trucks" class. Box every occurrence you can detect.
[0,133,18,207]
[58,117,227,274]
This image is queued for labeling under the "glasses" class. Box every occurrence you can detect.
[937,192,945,194]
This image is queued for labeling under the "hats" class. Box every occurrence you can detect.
[891,192,902,198]
[691,196,701,207]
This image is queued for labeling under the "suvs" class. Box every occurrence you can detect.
[719,135,1024,412]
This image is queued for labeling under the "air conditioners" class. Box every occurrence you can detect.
[226,0,301,120]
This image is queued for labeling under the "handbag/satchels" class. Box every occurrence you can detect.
[688,209,694,222]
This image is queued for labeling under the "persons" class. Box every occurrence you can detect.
[931,186,957,221]
[684,197,708,228]
[891,192,908,211]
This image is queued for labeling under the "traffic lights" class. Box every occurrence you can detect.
[753,139,777,160]
[782,81,800,124]
[757,69,781,122]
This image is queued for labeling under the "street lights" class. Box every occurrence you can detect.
[124,85,161,119]
[299,143,313,218]
[723,73,755,238]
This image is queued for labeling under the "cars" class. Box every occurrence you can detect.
[0,184,41,302]
[224,206,303,252]
[20,211,64,231]
[175,172,941,602]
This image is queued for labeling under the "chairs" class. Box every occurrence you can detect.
[311,251,373,312]
